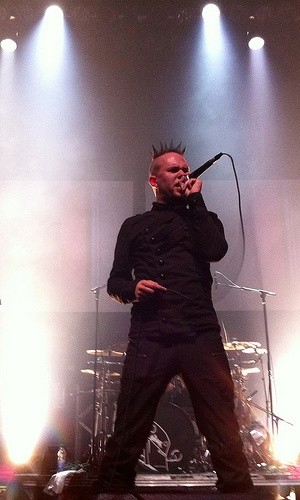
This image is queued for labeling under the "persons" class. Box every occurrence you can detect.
[93,137,276,500]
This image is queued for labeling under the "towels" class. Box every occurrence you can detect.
[43,469,85,496]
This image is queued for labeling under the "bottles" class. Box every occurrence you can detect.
[57,444,67,472]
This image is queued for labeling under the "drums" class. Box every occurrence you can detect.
[141,402,199,471]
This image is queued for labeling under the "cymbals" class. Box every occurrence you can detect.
[87,350,126,357]
[80,369,120,377]
[222,341,261,351]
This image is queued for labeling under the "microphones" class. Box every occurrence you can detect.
[214,272,218,289]
[185,152,223,181]
